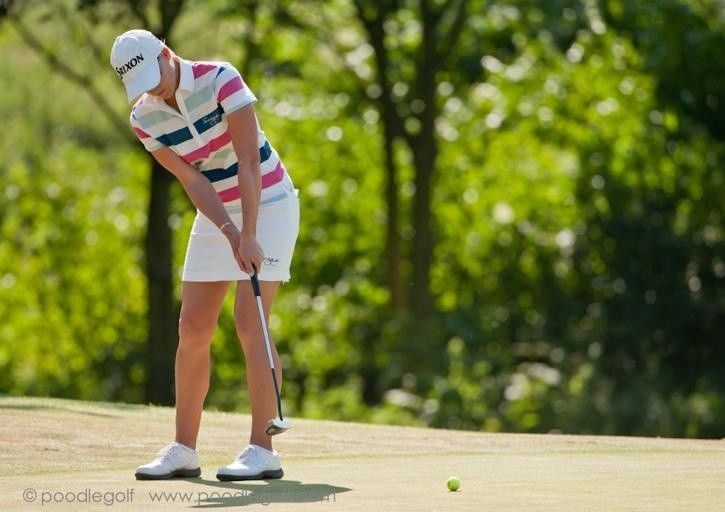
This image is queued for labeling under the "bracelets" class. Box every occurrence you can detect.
[219,222,230,231]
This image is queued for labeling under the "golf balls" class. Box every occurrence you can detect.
[447,478,459,491]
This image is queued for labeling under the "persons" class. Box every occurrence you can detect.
[110,30,300,480]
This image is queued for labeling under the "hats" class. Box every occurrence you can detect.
[110,29,165,104]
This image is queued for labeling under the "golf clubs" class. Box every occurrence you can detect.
[249,262,292,434]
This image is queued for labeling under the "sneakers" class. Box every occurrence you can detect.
[216,445,283,481]
[135,442,201,480]
